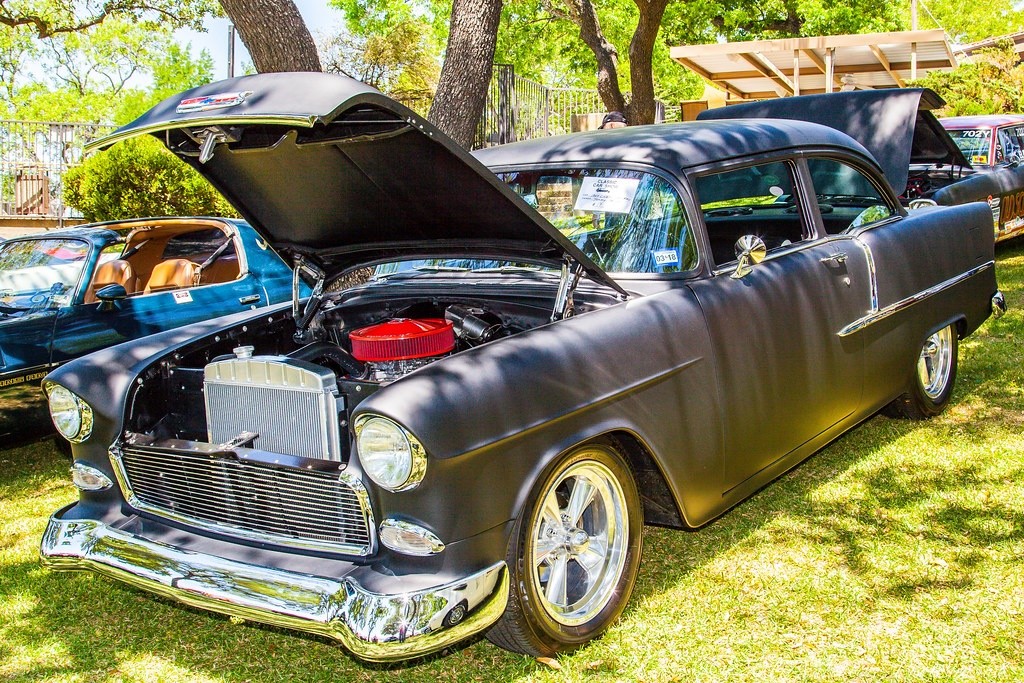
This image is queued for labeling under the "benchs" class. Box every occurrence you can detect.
[679,212,859,265]
[194,258,239,283]
[961,149,1015,160]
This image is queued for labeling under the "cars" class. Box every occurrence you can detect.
[809,114,1024,246]
[0,213,327,437]
[35,68,1009,664]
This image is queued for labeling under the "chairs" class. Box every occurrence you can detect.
[143,257,201,295]
[590,222,688,270]
[86,259,141,302]
